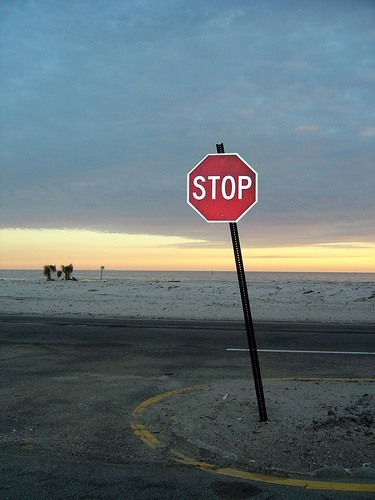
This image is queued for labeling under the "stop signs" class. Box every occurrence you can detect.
[186,153,258,223]
